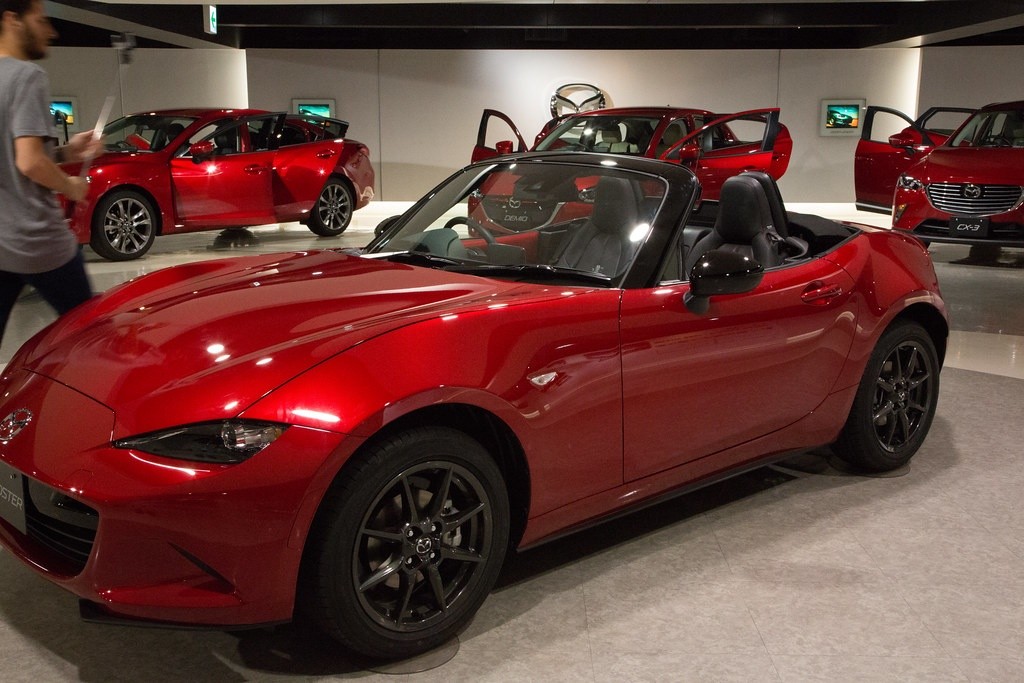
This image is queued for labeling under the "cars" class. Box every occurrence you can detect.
[851,101,1024,257]
[56,106,375,263]
[469,106,795,238]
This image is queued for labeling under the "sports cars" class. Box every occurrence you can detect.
[3,150,951,652]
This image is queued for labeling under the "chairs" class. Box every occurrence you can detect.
[213,123,238,153]
[654,124,684,160]
[547,167,656,281]
[593,123,640,152]
[684,167,797,284]
[165,121,191,155]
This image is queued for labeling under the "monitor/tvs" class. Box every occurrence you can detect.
[47,96,79,133]
[821,99,866,136]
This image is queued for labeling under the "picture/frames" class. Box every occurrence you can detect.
[44,93,80,134]
[818,97,868,140]
[290,97,336,125]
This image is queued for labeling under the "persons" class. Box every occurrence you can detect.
[0,0,95,361]
[150,122,196,157]
